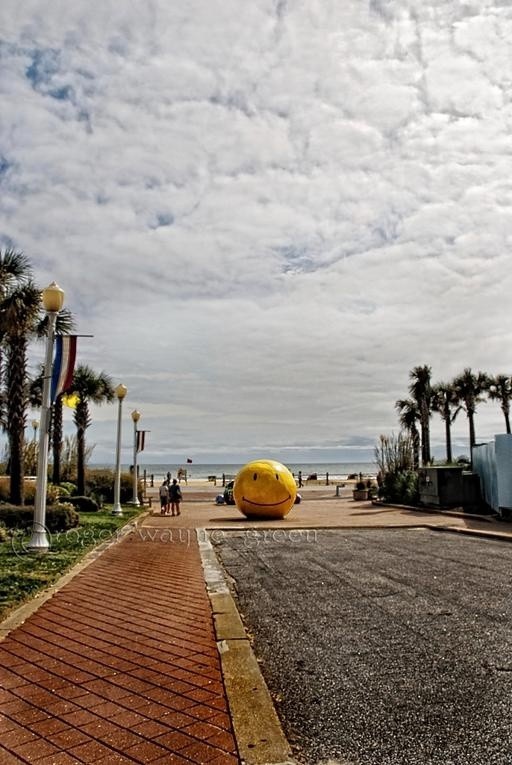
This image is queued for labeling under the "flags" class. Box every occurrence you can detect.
[52,335,76,403]
[136,431,145,452]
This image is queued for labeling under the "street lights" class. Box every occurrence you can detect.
[24,280,65,560]
[131,410,141,507]
[108,383,128,517]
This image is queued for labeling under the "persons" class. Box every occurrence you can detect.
[160,472,182,516]
[298,472,304,488]
